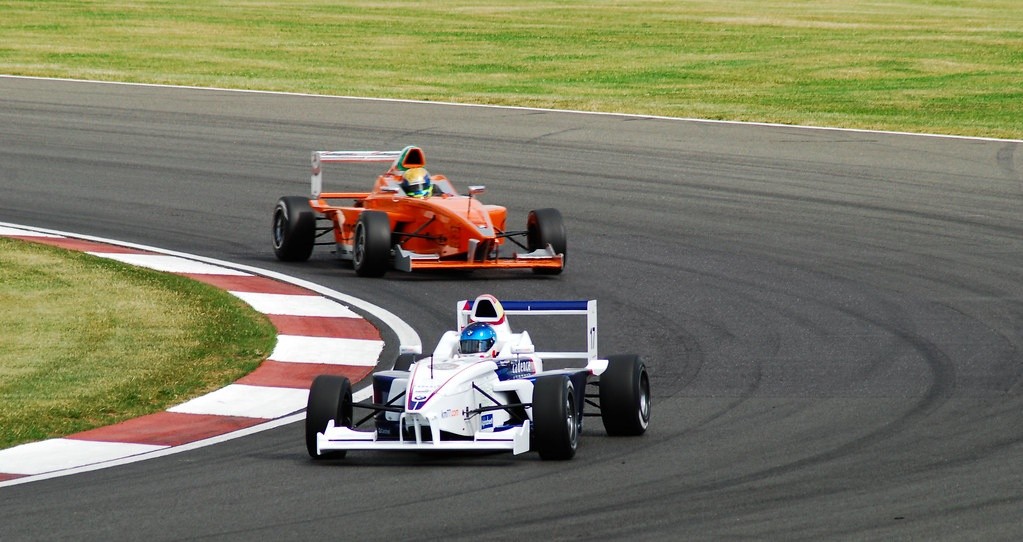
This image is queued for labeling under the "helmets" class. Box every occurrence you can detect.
[458,323,497,357]
[402,167,433,200]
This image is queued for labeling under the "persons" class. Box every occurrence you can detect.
[459,322,498,359]
[402,167,433,197]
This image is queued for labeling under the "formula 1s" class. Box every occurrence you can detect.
[305,292,651,460]
[276,146,568,280]
